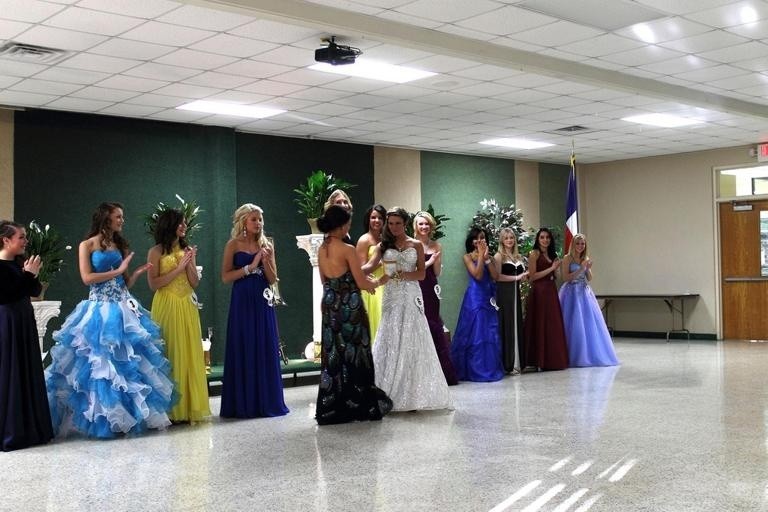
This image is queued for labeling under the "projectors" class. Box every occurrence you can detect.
[315,36,363,65]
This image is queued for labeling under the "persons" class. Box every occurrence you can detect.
[560,234,603,367]
[527,228,567,374]
[315,203,391,422]
[143,208,215,425]
[563,161,579,255]
[360,206,435,412]
[0,221,56,451]
[221,202,288,418]
[353,203,387,347]
[493,228,530,374]
[72,203,153,439]
[461,227,498,382]
[321,190,356,248]
[410,210,458,387]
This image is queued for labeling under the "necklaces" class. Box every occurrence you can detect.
[399,247,405,252]
[102,229,114,249]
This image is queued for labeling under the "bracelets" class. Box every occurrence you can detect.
[485,259,491,265]
[244,265,250,276]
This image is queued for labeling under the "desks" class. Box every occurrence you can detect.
[593,292,698,342]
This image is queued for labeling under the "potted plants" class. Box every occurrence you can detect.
[293,168,360,235]
[23,218,72,302]
